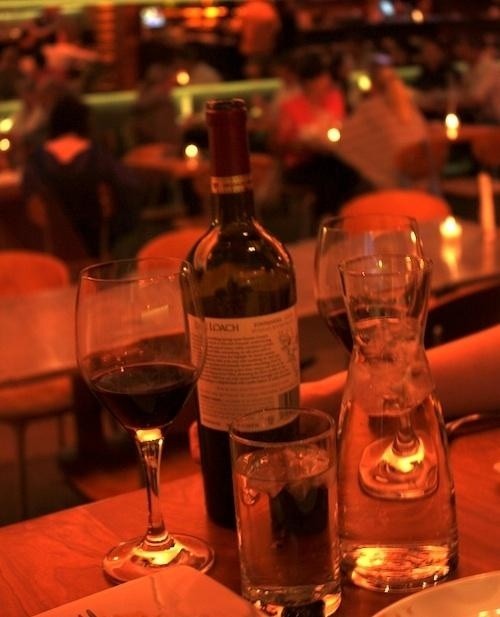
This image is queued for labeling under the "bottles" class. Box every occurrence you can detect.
[180,97,300,529]
[335,254,461,594]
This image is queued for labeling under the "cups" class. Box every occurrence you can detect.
[230,404,343,617]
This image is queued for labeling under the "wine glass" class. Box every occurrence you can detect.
[315,212,442,498]
[75,257,218,585]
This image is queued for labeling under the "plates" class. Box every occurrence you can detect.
[371,569,499,617]
[30,563,272,615]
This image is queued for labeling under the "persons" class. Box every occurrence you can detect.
[3,0,499,258]
